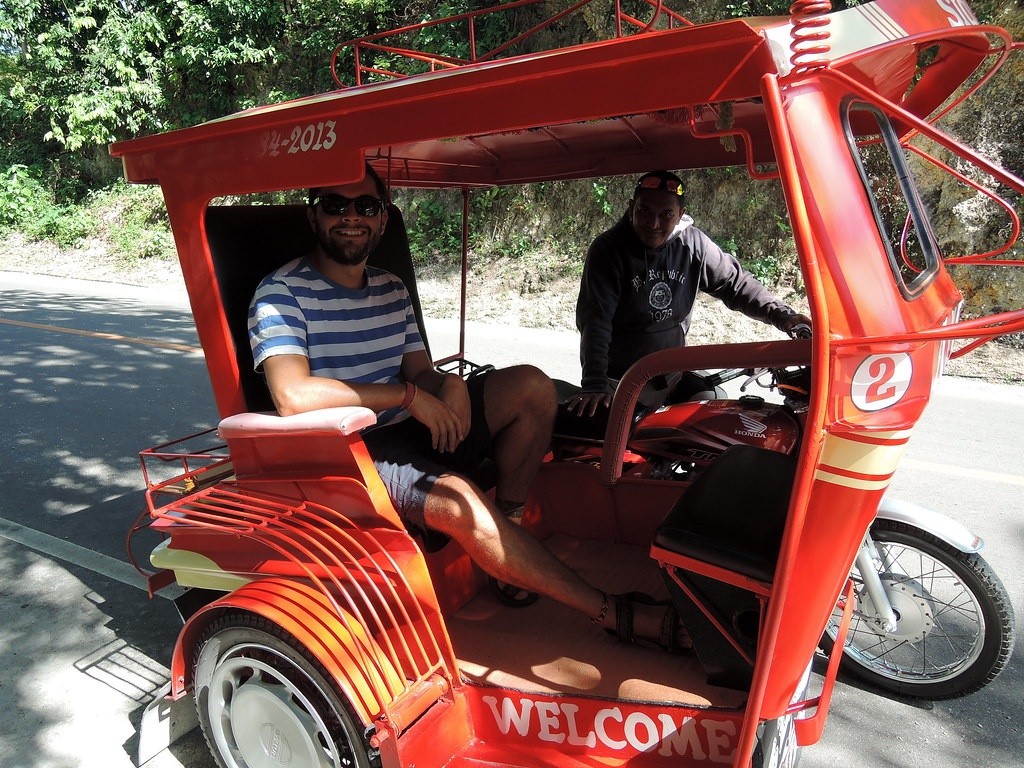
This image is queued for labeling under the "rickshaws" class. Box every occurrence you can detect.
[127,1,1022,767]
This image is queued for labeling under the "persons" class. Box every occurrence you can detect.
[562,169,812,416]
[247,160,695,656]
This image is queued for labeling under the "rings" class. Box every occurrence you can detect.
[578,397,582,400]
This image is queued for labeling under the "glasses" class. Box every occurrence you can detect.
[310,193,383,217]
[639,175,686,196]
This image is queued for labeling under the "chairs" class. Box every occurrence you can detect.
[206,202,550,546]
[645,445,802,691]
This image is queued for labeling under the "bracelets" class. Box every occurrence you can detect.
[401,382,417,410]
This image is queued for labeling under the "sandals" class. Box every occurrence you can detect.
[603,591,696,655]
[487,564,540,607]
